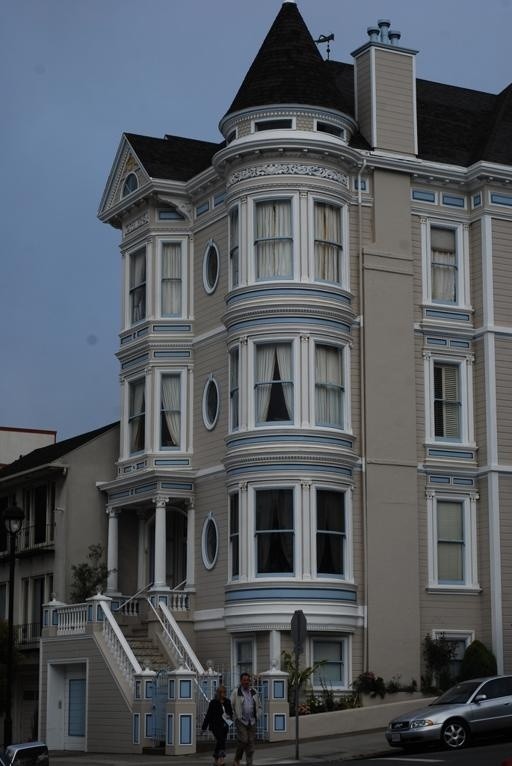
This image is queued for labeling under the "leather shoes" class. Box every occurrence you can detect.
[247,761,252,766]
[233,759,241,766]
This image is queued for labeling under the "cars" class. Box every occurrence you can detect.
[385,673,512,747]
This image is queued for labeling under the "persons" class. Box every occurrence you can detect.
[200,686,233,766]
[230,673,262,765]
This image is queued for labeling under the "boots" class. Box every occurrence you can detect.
[220,757,226,766]
[214,758,218,766]
